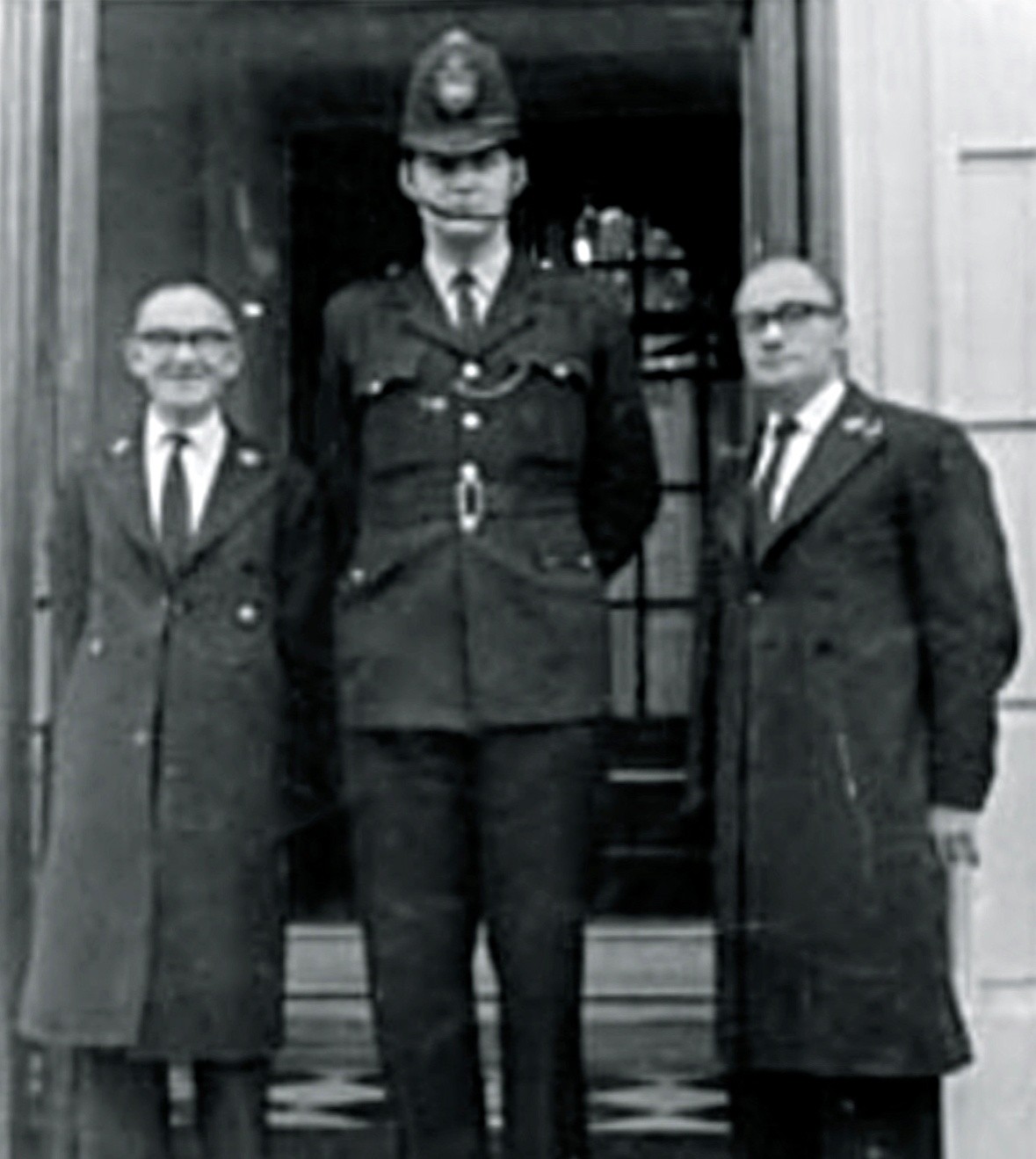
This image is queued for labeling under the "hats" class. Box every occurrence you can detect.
[397,25,522,157]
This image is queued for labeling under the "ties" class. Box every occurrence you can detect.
[750,417,799,550]
[161,433,189,594]
[453,272,478,341]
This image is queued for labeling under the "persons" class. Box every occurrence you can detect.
[693,256,1015,1159]
[324,32,664,1159]
[10,271,320,1159]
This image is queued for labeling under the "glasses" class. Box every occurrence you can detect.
[135,326,233,346]
[738,300,839,330]
